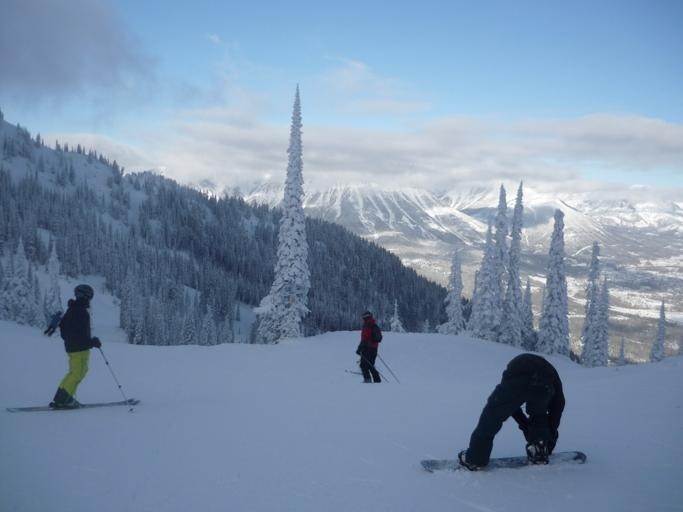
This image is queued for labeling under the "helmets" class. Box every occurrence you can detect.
[74,284,94,297]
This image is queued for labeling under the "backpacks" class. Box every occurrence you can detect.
[365,323,382,343]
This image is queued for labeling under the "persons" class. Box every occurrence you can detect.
[456,352,566,472]
[44,310,62,337]
[49,285,101,408]
[356,311,382,383]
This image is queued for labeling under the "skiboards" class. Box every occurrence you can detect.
[5,398,140,412]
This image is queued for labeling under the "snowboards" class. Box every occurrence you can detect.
[421,451,586,474]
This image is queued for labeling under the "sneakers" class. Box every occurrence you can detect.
[522,440,550,465]
[456,449,487,472]
[53,388,79,407]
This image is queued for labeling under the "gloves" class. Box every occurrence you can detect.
[91,337,102,348]
